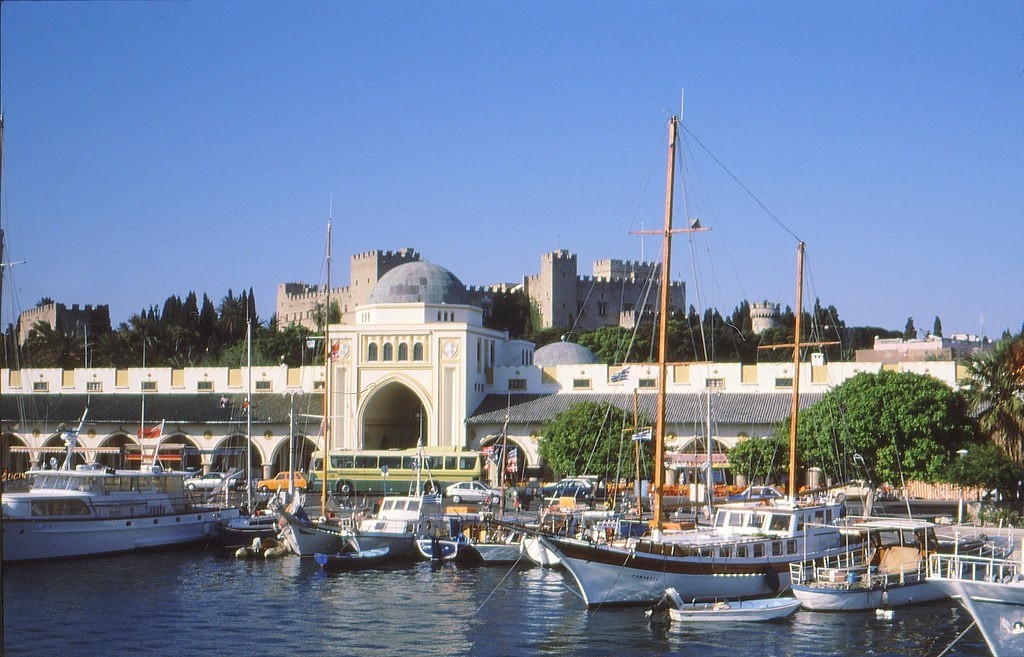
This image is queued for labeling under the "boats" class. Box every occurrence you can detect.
[646,586,804,626]
[787,511,1017,614]
[1,406,240,564]
[340,402,448,558]
[924,553,1024,656]
[314,546,393,569]
[416,513,538,560]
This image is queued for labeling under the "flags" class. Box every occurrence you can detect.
[505,449,518,473]
[482,446,502,473]
[631,430,651,440]
[138,423,162,439]
[330,341,339,360]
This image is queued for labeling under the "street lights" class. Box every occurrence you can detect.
[958,450,971,528]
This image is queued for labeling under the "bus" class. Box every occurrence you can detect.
[308,445,482,495]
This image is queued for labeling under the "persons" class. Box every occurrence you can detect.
[219,393,228,408]
[243,398,248,415]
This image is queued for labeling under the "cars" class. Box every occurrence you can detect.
[536,477,595,501]
[183,471,237,491]
[257,470,308,494]
[579,477,607,495]
[730,483,784,501]
[444,480,501,504]
[982,479,1022,502]
[832,477,886,502]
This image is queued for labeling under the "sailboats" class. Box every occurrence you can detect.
[271,212,370,557]
[469,386,668,565]
[211,316,295,553]
[484,108,887,613]
